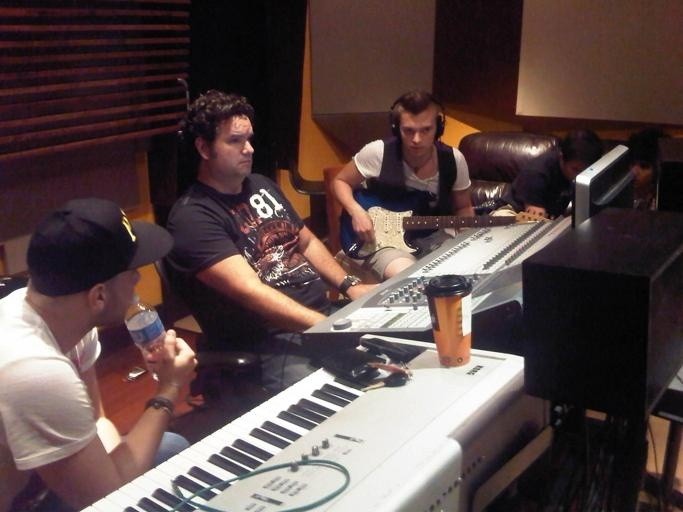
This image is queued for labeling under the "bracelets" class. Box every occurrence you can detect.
[336,272,363,294]
[143,396,178,413]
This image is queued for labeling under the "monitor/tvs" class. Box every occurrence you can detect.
[572,144,636,230]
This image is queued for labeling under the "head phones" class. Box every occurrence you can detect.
[388,89,446,139]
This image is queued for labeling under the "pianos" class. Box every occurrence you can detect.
[77,336,549,512]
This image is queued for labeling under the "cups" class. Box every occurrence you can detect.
[425,275,473,367]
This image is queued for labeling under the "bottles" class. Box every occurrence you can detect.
[123,292,166,382]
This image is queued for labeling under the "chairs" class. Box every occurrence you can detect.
[457,130,557,211]
[152,256,274,416]
[323,165,368,299]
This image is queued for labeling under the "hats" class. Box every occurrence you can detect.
[24,196,181,299]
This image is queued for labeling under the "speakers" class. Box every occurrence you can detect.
[522,206,682,420]
[655,136,683,212]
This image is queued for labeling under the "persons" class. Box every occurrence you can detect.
[619,127,674,213]
[159,91,387,413]
[327,91,476,272]
[1,195,200,512]
[490,129,603,223]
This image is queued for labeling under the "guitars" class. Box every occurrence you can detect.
[357,205,555,259]
